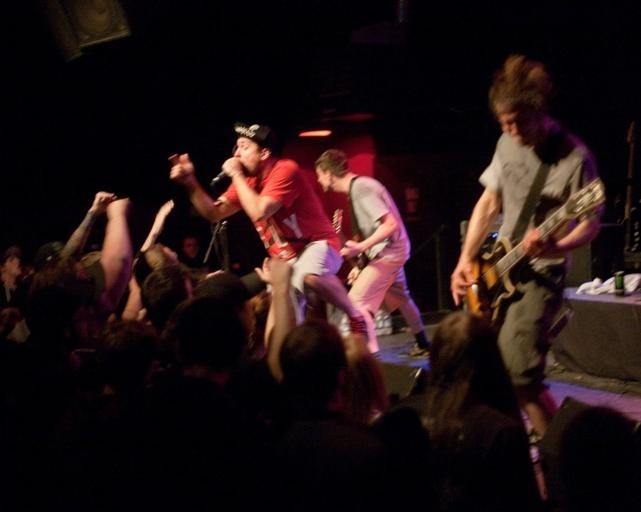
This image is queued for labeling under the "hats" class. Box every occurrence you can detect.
[234,124,276,151]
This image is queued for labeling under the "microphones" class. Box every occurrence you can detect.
[210,170,227,187]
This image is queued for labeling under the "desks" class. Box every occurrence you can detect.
[554,287,641,382]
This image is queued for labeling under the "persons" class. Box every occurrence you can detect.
[450,52,607,438]
[316,148,431,359]
[1,189,641,512]
[167,124,382,356]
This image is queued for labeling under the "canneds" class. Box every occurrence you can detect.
[614,271,624,296]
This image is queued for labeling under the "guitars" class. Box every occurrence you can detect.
[464,176,605,330]
[331,209,369,270]
